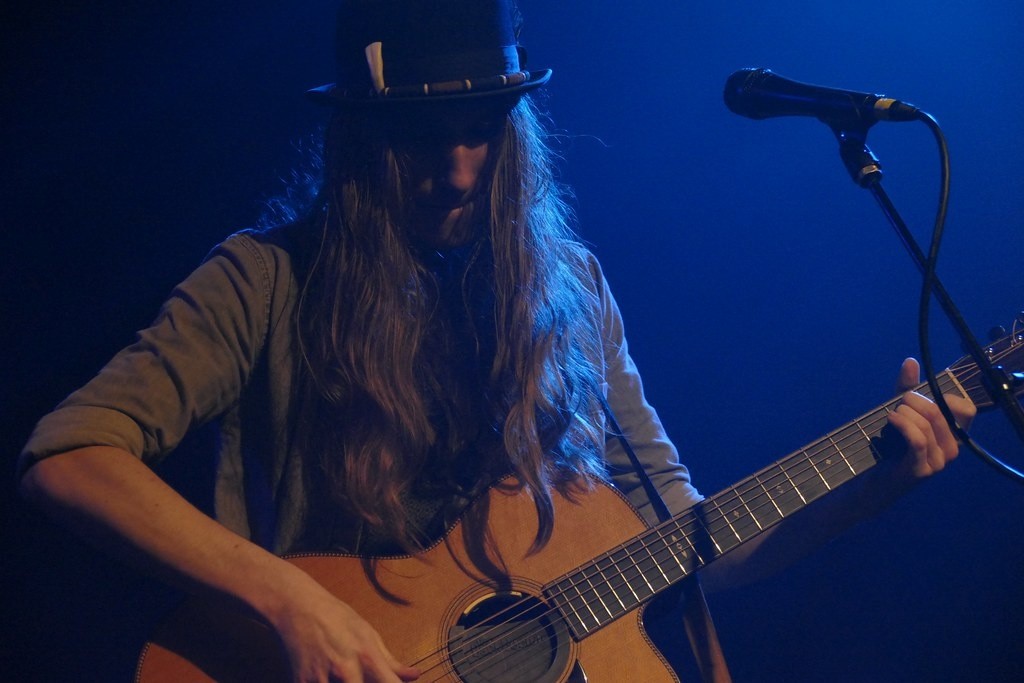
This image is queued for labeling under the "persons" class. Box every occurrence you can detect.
[9,0,976,683]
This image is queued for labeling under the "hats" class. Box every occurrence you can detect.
[307,0,552,99]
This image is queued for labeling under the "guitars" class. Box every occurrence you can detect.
[131,313,1024,682]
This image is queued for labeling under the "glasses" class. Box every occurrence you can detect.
[380,100,512,148]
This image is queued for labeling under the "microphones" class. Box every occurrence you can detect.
[724,67,921,121]
